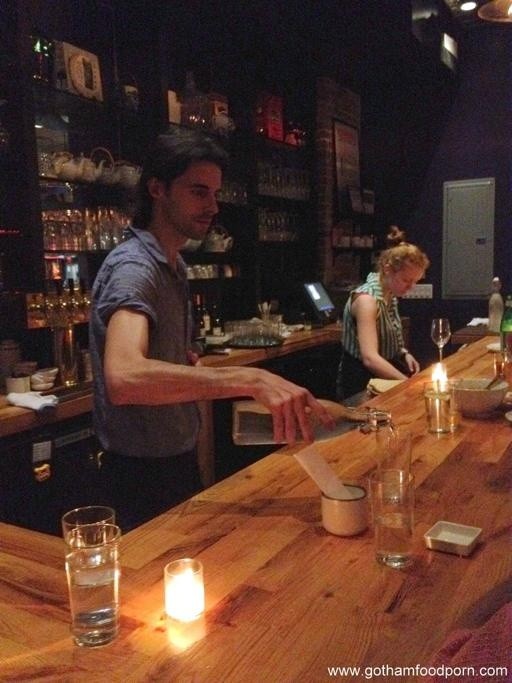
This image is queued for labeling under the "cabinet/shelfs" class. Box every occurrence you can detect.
[334,225,380,294]
[2,319,421,543]
[1,85,316,386]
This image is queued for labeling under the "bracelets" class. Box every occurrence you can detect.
[400,349,410,365]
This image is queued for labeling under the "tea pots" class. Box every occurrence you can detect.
[37,134,143,184]
[204,228,232,253]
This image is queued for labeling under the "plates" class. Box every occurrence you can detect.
[424,521,482,556]
[28,366,59,392]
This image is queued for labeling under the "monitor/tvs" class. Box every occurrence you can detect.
[303,281,338,320]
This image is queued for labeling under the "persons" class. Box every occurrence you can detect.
[329,223,433,414]
[88,124,338,538]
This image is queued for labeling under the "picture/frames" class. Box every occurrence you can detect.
[332,119,365,219]
[348,184,374,216]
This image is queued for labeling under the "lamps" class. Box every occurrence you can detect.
[477,0,510,24]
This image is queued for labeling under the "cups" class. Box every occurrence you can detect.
[320,484,369,537]
[5,375,30,395]
[163,559,205,621]
[373,428,417,573]
[60,505,121,650]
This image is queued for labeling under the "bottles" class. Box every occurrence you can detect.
[488,277,512,357]
[231,397,393,446]
[193,293,225,337]
[34,278,83,324]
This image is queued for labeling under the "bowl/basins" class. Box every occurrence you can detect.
[451,379,509,417]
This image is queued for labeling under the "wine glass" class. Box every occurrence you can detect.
[430,318,452,376]
